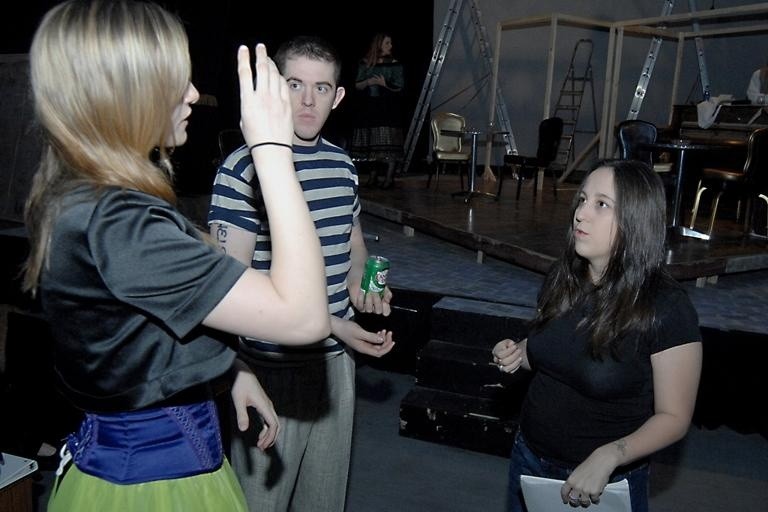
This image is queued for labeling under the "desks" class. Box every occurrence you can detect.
[440,129,510,204]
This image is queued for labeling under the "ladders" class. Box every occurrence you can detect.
[613,0,712,162]
[393,0,527,180]
[551,39,602,184]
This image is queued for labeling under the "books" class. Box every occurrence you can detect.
[517,473,634,512]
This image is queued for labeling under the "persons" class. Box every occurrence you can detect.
[747,64,767,104]
[205,39,399,512]
[346,30,408,192]
[490,157,709,511]
[15,1,336,510]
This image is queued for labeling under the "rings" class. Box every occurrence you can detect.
[578,498,590,505]
[566,491,582,507]
[498,364,504,371]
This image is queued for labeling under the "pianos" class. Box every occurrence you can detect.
[651,103,766,244]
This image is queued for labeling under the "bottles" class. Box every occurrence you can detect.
[703,84,710,102]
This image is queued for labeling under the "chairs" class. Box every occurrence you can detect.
[494,116,564,202]
[425,112,469,193]
[617,119,768,242]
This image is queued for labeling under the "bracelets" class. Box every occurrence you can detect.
[248,141,295,154]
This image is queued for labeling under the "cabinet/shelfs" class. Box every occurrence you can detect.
[672,104,768,128]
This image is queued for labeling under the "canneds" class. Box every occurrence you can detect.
[359,254,391,304]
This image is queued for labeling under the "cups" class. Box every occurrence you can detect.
[758,95,765,103]
